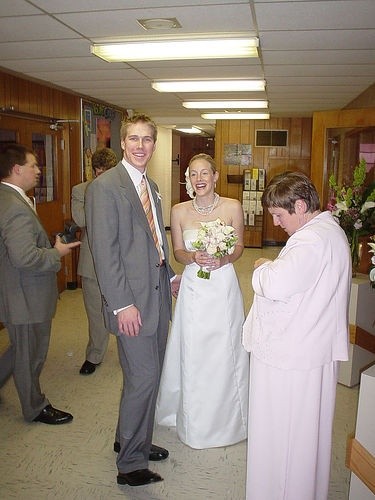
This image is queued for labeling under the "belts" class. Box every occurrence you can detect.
[159,260,166,267]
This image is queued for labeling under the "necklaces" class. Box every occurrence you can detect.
[191,193,220,216]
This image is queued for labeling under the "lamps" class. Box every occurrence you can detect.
[89,37,260,64]
[200,111,270,120]
[182,98,269,109]
[151,78,267,93]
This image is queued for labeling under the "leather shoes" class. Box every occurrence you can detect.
[117,468,164,486]
[113,440,170,461]
[79,359,103,375]
[31,404,73,425]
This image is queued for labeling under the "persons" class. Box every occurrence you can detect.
[156,153,249,448]
[0,147,121,425]
[86,115,183,488]
[240,170,349,500]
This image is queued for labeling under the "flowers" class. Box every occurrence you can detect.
[191,217,238,279]
[323,158,375,230]
[367,235,375,289]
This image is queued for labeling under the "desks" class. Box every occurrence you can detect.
[349,364,375,500]
[337,273,375,387]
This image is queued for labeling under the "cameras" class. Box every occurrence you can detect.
[52,220,80,245]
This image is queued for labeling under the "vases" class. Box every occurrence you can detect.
[347,242,363,277]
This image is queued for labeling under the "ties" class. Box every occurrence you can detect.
[138,175,162,267]
